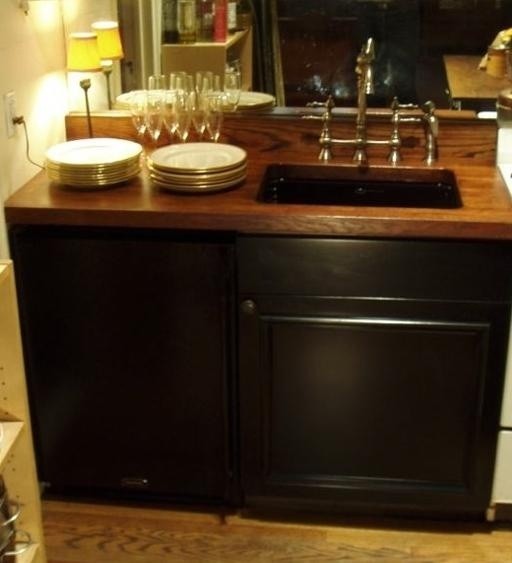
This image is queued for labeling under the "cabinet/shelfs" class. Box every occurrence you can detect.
[161,24,253,95]
[5,235,512,530]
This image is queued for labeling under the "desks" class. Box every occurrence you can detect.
[445,54,512,110]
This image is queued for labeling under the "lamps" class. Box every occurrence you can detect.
[92,20,124,110]
[67,34,104,138]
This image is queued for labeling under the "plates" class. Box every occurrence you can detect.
[45,138,142,190]
[146,142,250,196]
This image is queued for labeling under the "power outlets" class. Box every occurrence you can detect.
[4,90,18,139]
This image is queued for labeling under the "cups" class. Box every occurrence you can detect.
[126,68,247,140]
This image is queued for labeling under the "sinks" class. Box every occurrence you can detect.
[256,162,462,210]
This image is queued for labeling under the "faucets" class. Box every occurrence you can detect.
[301,36,421,167]
